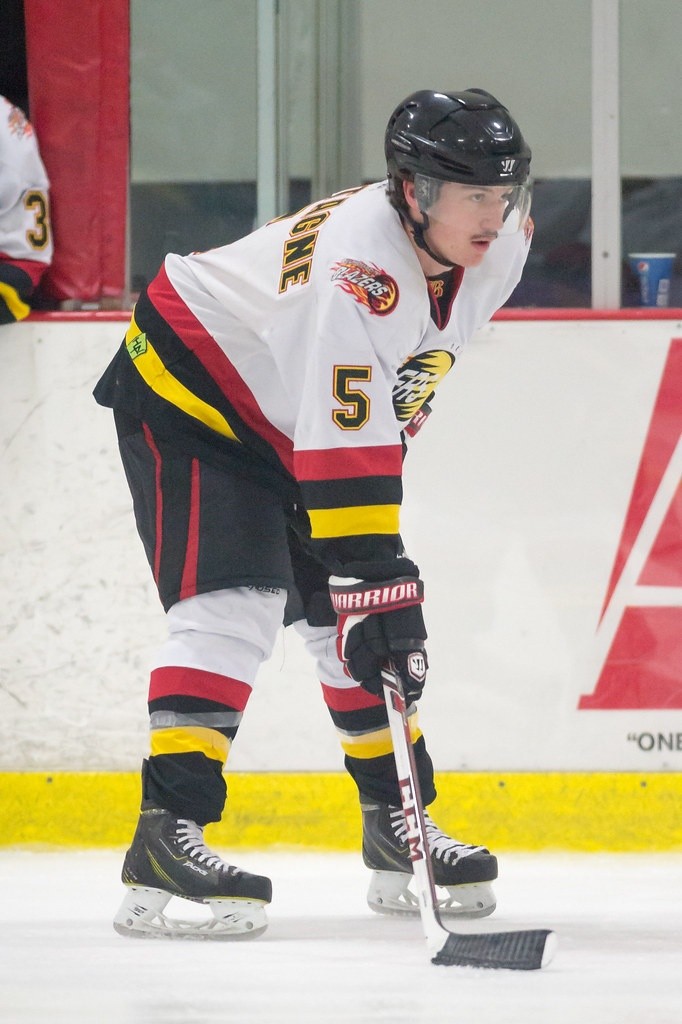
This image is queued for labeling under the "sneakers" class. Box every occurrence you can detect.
[361,794,499,922]
[112,804,272,943]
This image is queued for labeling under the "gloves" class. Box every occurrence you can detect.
[323,557,429,713]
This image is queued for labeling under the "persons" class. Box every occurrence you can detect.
[91,89,532,940]
[0,92,54,325]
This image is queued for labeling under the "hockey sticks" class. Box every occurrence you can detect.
[380,659,560,973]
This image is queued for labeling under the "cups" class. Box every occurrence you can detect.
[628,251,676,309]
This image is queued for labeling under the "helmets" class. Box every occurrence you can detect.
[384,89,535,211]
